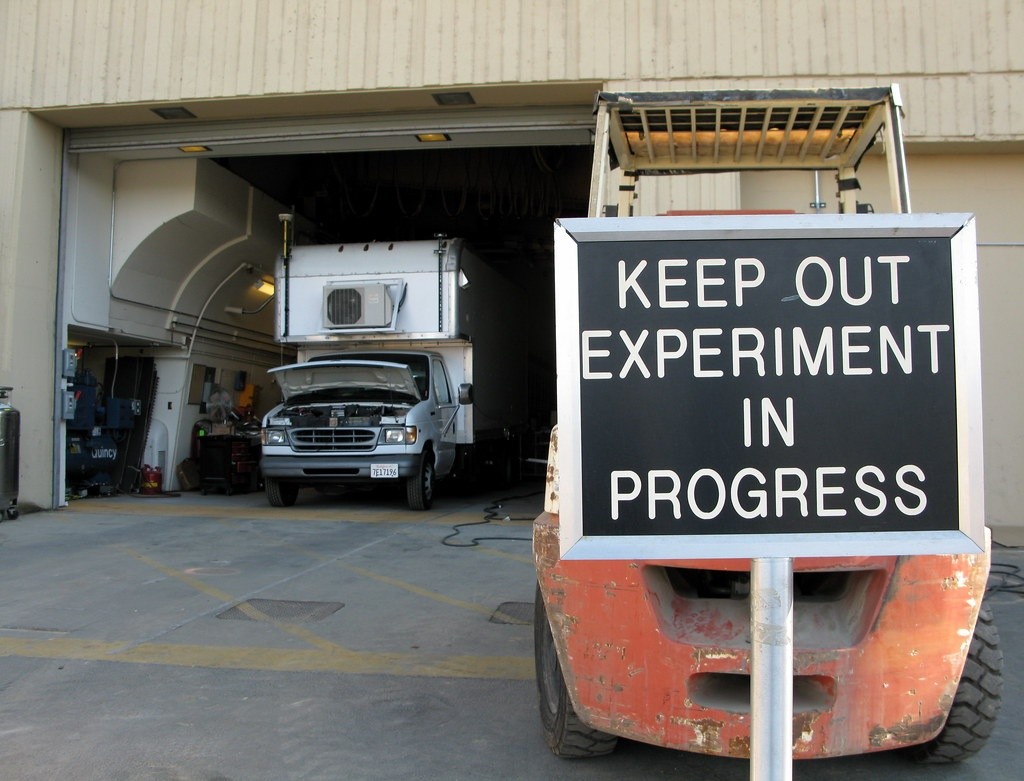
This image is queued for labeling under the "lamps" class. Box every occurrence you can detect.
[255,279,274,295]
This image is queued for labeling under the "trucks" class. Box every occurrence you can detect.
[260,213,530,512]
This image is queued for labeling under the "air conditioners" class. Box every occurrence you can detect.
[321,283,392,328]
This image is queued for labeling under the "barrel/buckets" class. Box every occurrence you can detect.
[140,470,162,494]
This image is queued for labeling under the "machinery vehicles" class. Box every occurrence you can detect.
[527,85,1005,768]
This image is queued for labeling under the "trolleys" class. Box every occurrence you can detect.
[200,435,253,496]
